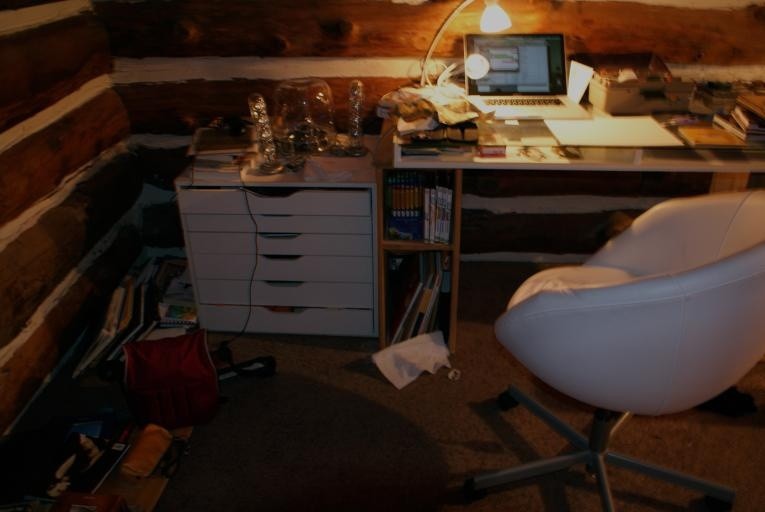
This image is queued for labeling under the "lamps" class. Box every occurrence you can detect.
[421,0,512,86]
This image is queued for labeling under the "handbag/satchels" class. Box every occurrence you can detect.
[119,424,179,478]
[120,326,221,428]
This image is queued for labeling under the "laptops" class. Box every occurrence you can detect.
[463,32,591,120]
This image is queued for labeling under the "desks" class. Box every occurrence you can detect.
[371,114,765,356]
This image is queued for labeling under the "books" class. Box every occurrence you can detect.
[676,74,764,156]
[40,240,202,512]
[385,173,456,347]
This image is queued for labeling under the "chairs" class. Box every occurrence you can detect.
[460,190,765,512]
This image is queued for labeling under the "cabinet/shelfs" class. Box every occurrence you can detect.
[174,134,379,339]
